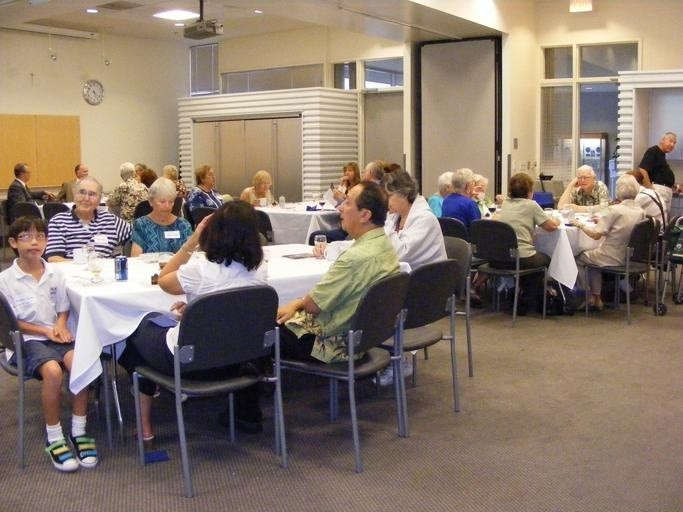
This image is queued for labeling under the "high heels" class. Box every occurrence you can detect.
[141,430,154,447]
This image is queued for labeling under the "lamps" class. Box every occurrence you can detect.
[569,0,593,12]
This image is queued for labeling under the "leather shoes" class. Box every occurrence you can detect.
[594,300,603,310]
[577,300,595,310]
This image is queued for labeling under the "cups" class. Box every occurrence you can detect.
[486,205,497,216]
[558,208,571,225]
[313,234,327,260]
[544,208,552,221]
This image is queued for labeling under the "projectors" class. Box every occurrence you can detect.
[182,19,224,39]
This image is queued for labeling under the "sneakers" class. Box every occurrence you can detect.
[45,435,79,472]
[372,357,415,387]
[220,408,264,434]
[68,430,99,468]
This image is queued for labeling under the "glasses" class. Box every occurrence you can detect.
[17,231,45,241]
[76,189,98,198]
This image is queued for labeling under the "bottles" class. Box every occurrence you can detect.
[279,193,285,208]
[115,255,128,280]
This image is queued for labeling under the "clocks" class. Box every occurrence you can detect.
[83,80,103,106]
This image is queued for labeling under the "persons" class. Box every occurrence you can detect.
[428,172,456,217]
[164,165,187,216]
[118,200,269,450]
[240,170,278,206]
[7,162,57,225]
[135,163,149,175]
[57,164,89,202]
[490,173,561,315]
[442,169,490,308]
[312,169,447,386]
[570,174,647,311]
[638,168,655,190]
[364,159,401,180]
[218,181,401,433]
[363,162,385,189]
[0,215,99,471]
[138,168,158,190]
[131,177,195,254]
[135,164,158,188]
[46,175,132,262]
[338,163,362,196]
[639,132,683,224]
[107,162,149,225]
[626,168,668,260]
[557,165,609,213]
[471,174,506,218]
[187,165,225,230]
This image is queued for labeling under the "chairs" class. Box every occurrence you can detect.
[134,200,153,219]
[631,216,680,294]
[132,286,287,497]
[438,217,496,312]
[335,260,461,438]
[3,200,13,248]
[0,291,111,469]
[412,237,474,387]
[43,203,70,221]
[309,231,331,246]
[465,220,547,328]
[272,273,408,473]
[585,219,660,325]
[182,202,195,232]
